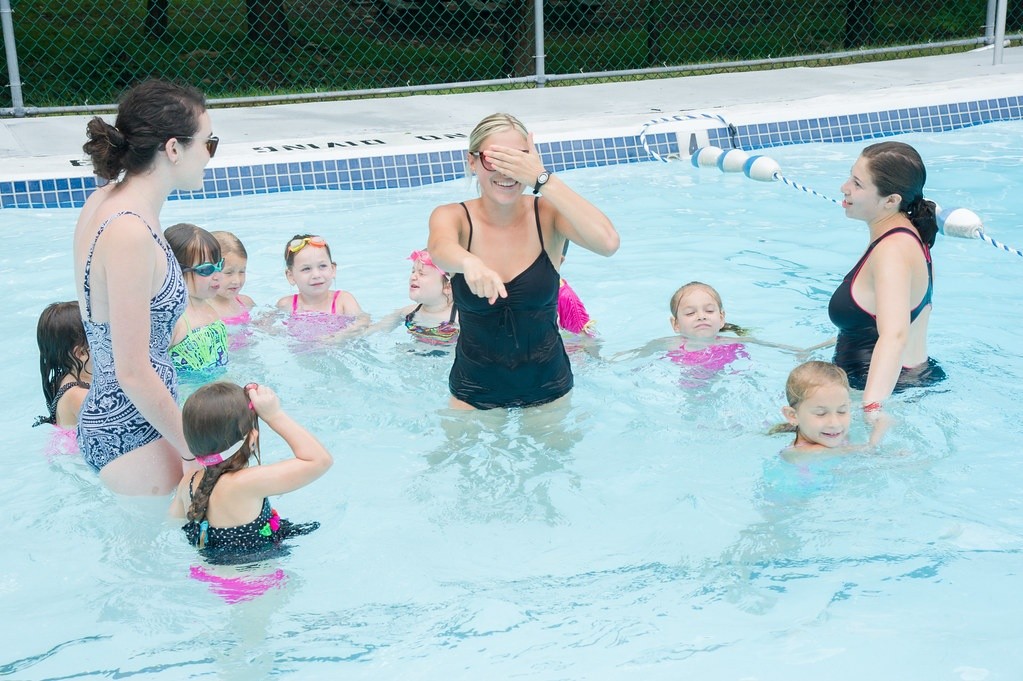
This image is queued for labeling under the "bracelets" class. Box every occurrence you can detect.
[182,456,195,461]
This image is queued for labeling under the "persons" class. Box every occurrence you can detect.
[557,238,597,334]
[74,81,220,496]
[206,230,255,313]
[427,113,620,410]
[163,223,227,368]
[171,383,334,535]
[277,235,362,313]
[35,301,92,429]
[784,360,852,446]
[669,282,726,339]
[405,248,459,320]
[828,141,938,399]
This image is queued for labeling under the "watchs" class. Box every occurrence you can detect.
[533,170,551,194]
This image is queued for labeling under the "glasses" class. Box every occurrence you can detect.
[181,256,226,277]
[468,150,529,172]
[158,133,219,158]
[410,252,452,282]
[286,235,331,264]
